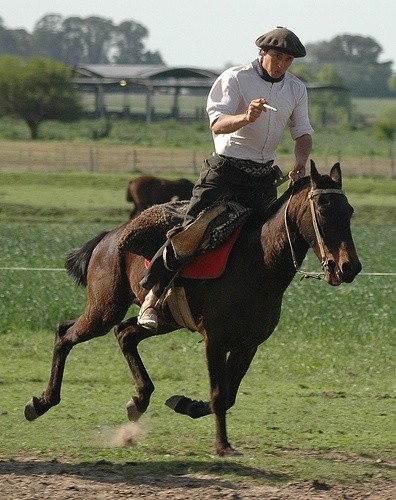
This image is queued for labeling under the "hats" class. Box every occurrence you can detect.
[255,27,306,58]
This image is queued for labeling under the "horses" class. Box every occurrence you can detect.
[24,158,362,457]
[126,175,195,222]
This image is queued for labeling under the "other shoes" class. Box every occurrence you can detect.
[137,308,158,330]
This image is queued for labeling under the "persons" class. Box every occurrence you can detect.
[137,27,312,330]
